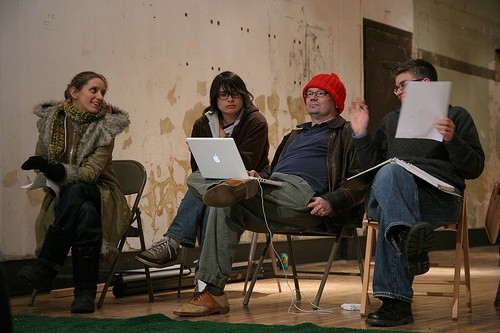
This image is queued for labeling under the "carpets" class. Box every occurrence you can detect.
[11,313,419,333]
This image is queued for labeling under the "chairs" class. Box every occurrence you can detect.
[28,160,153,309]
[178,188,472,320]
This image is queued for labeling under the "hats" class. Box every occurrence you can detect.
[303,73,346,114]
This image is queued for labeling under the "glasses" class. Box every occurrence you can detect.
[217,90,242,100]
[394,78,424,95]
[303,91,329,99]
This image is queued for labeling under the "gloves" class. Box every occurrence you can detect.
[20,155,63,178]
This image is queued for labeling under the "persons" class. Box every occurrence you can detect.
[348,59,485,327]
[134,70,270,292]
[173,73,368,317]
[19,71,132,314]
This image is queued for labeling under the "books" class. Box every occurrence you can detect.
[347,157,462,197]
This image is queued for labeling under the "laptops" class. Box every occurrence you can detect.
[185,137,283,187]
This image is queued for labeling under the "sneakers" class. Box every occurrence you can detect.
[203,179,258,207]
[134,240,181,268]
[70,285,96,311]
[18,262,56,293]
[174,290,229,317]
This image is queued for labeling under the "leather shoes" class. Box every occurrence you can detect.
[366,296,414,326]
[400,221,433,276]
